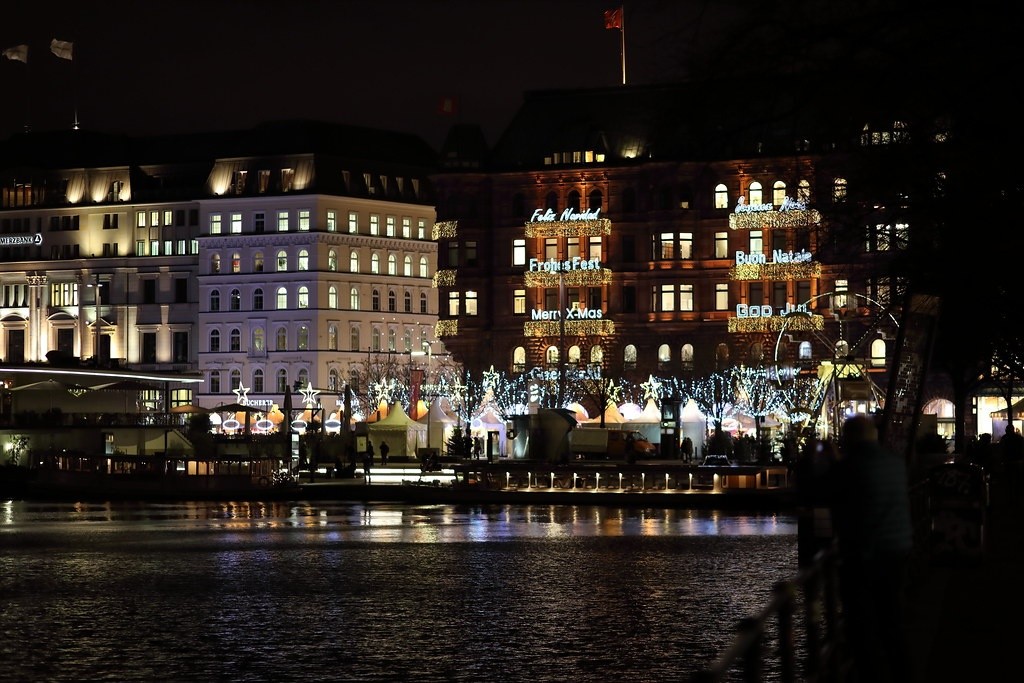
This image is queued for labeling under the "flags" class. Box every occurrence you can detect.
[3,45,28,64]
[604,5,624,31]
[50,38,72,61]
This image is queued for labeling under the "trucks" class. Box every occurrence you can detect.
[571,427,658,464]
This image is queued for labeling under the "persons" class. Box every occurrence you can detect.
[299,438,389,468]
[625,432,635,462]
[776,416,1024,683]
[681,438,693,462]
[135,393,165,412]
[474,438,481,460]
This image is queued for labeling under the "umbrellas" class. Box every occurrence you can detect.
[283,385,293,432]
[8,379,93,412]
[88,379,167,413]
[167,403,271,435]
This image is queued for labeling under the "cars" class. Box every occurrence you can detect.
[698,454,732,485]
[246,468,298,488]
[548,465,583,489]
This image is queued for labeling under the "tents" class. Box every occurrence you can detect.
[209,399,782,457]
[989,398,1024,443]
